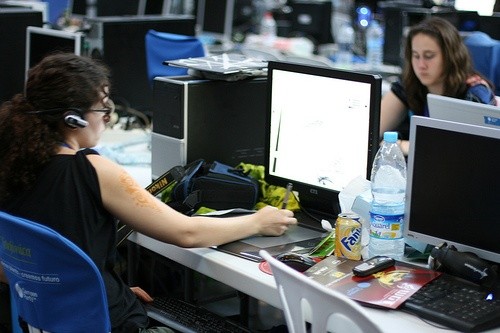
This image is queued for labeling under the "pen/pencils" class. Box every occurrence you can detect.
[282,183,294,210]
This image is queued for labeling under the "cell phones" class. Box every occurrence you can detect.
[352,255,395,276]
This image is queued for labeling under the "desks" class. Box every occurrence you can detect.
[120,129,500,333]
[461,30,500,94]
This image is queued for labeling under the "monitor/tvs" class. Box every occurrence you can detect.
[196,0,234,53]
[402,94,500,264]
[24,26,85,92]
[263,60,383,232]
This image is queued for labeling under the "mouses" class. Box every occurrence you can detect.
[277,252,316,271]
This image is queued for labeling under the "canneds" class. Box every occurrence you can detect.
[334,212,364,260]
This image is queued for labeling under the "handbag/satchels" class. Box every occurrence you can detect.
[173,158,260,210]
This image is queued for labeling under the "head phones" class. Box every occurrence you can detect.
[62,108,88,128]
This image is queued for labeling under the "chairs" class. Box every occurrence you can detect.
[0,211,111,333]
[144,28,204,84]
[259,249,385,333]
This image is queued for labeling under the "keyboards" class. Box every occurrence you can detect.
[401,276,500,333]
[142,296,256,333]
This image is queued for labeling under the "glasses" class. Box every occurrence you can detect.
[79,99,114,115]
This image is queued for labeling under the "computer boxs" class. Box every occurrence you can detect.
[149,75,269,187]
[0,8,43,105]
[80,14,196,120]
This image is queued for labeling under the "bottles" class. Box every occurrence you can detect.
[369,131,408,261]
[365,20,384,71]
[260,12,277,38]
[270,36,315,59]
[335,21,355,65]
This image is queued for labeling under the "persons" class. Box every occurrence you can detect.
[0,54,297,333]
[380,18,498,154]
